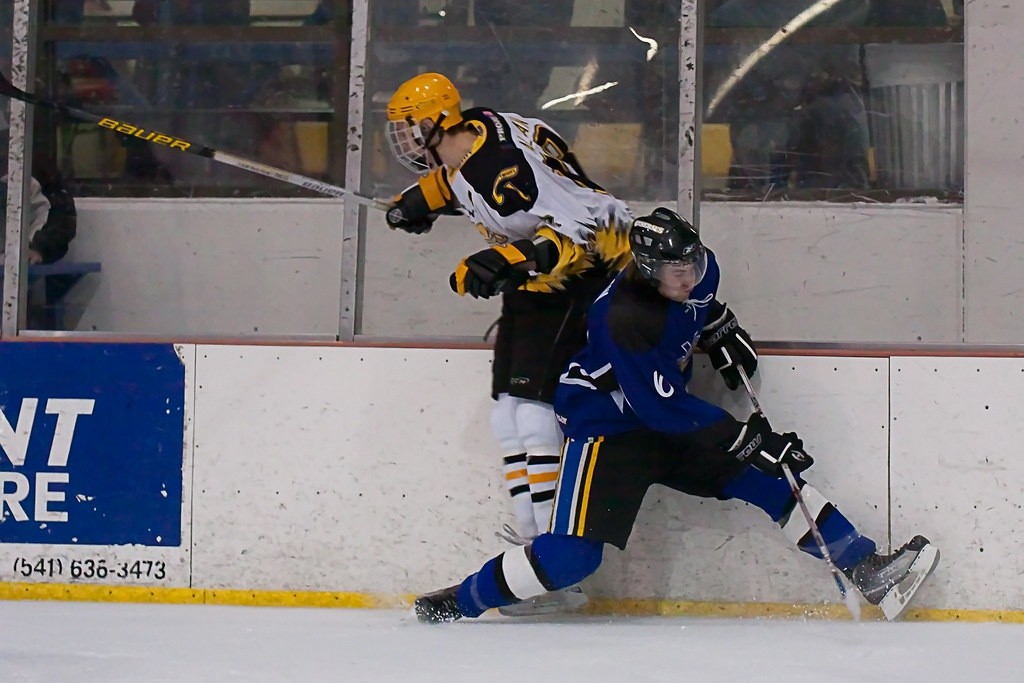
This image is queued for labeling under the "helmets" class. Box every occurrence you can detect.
[386,73,463,130]
[629,207,702,279]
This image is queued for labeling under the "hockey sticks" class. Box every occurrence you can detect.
[0,73,392,213]
[734,363,863,622]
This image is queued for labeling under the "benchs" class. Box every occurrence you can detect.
[1,0,679,203]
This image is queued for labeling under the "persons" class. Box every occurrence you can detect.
[385,72,636,617]
[0,126,76,331]
[415,205,940,626]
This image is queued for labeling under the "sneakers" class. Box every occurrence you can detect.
[495,524,589,616]
[842,534,941,622]
[400,585,463,625]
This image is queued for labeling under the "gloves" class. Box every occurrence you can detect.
[697,302,758,391]
[389,185,439,234]
[727,413,814,476]
[449,239,537,299]
